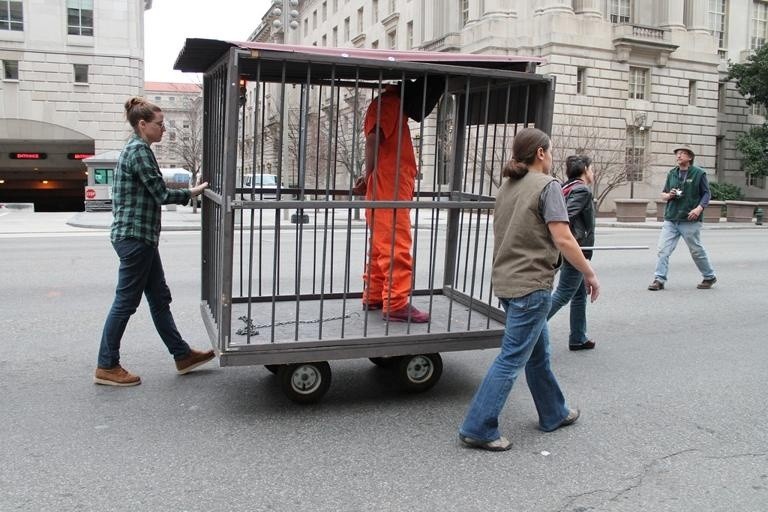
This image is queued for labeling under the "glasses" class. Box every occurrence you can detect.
[148,122,165,128]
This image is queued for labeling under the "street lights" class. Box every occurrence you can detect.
[630,112,647,198]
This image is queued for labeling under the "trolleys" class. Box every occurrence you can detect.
[181,48,555,404]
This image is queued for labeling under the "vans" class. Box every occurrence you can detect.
[242,172,285,202]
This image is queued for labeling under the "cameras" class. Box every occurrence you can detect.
[675,188,683,200]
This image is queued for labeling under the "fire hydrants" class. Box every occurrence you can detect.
[755,207,765,226]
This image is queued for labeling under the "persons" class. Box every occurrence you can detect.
[351,75,445,324]
[648,144,716,290]
[458,128,599,451]
[547,155,595,350]
[93,98,215,386]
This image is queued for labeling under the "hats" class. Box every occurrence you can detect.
[673,144,695,155]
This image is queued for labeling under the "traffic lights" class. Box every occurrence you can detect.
[239,79,247,106]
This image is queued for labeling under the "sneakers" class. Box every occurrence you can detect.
[648,279,664,290]
[92,364,141,387]
[173,350,216,374]
[382,303,430,324]
[363,300,383,310]
[561,407,580,425]
[568,339,595,351]
[460,430,513,451]
[697,277,717,289]
